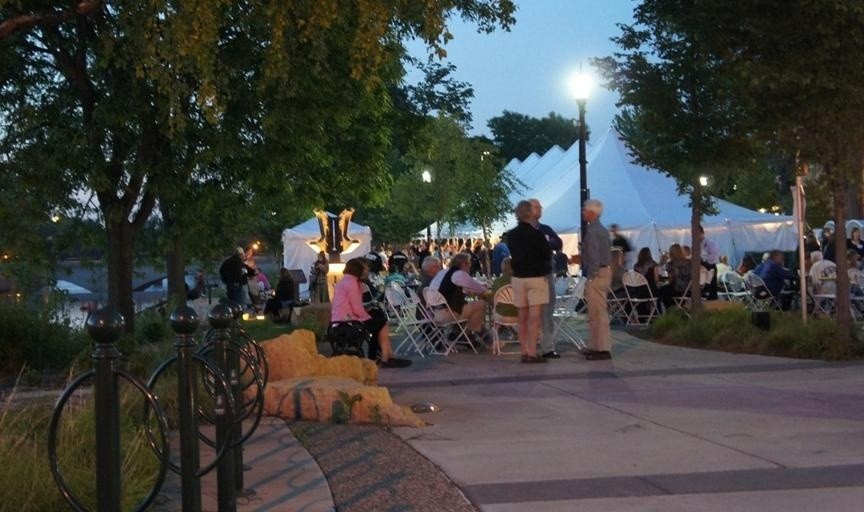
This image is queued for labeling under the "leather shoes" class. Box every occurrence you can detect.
[378,358,411,368]
[525,351,560,363]
[586,353,611,359]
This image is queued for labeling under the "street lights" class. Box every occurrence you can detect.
[568,60,598,247]
[421,171,437,243]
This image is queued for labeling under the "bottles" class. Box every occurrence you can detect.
[476,271,503,284]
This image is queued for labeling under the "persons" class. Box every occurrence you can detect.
[308,200,568,368]
[580,201,863,359]
[187,246,295,324]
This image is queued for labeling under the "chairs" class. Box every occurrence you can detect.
[424,291,478,357]
[806,275,837,323]
[622,271,659,330]
[387,282,434,359]
[743,271,785,313]
[846,267,864,324]
[717,270,748,306]
[672,280,693,322]
[552,276,587,351]
[406,288,451,352]
[491,284,524,357]
[607,279,631,327]
[810,259,837,286]
